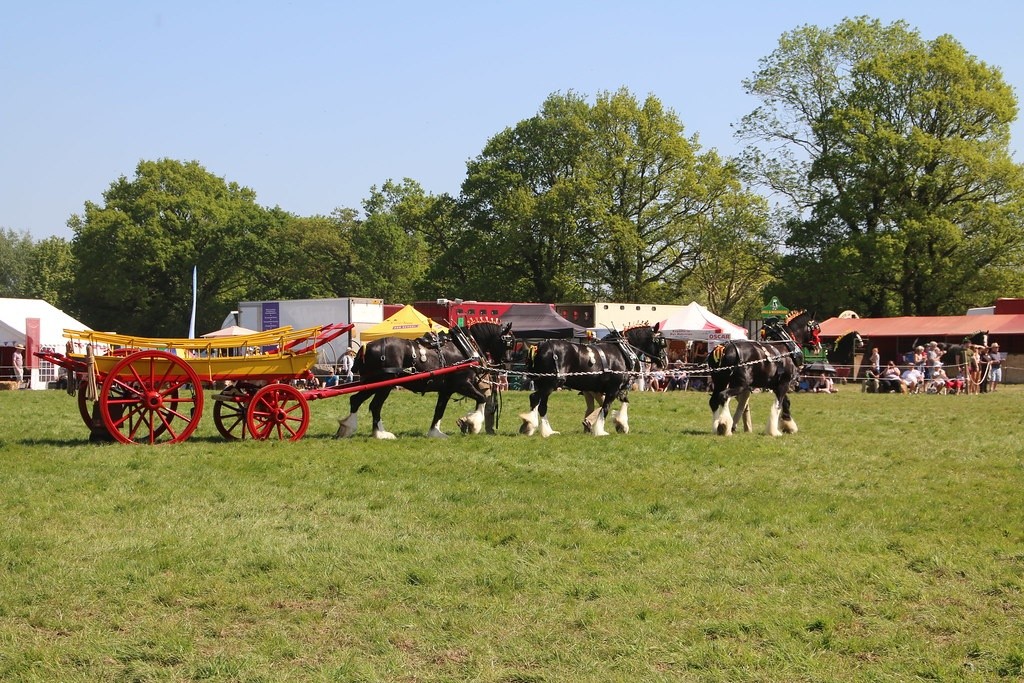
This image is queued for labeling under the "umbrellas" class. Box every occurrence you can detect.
[200,326,260,336]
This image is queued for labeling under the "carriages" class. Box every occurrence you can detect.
[32,306,823,445]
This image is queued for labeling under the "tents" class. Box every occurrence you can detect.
[0,298,110,389]
[360,304,449,350]
[653,300,748,344]
[498,302,588,341]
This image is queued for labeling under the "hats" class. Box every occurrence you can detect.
[15,344,25,350]
[673,360,684,366]
[988,342,999,348]
[906,363,916,366]
[928,341,938,347]
[932,361,943,368]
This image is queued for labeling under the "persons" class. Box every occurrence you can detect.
[246,346,263,356]
[12,344,25,383]
[628,341,708,391]
[867,340,1006,394]
[790,373,838,393]
[321,367,338,389]
[343,347,354,383]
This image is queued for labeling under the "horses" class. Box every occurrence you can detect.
[825,331,864,364]
[518,321,668,438]
[706,309,822,437]
[333,317,516,438]
[913,330,990,365]
